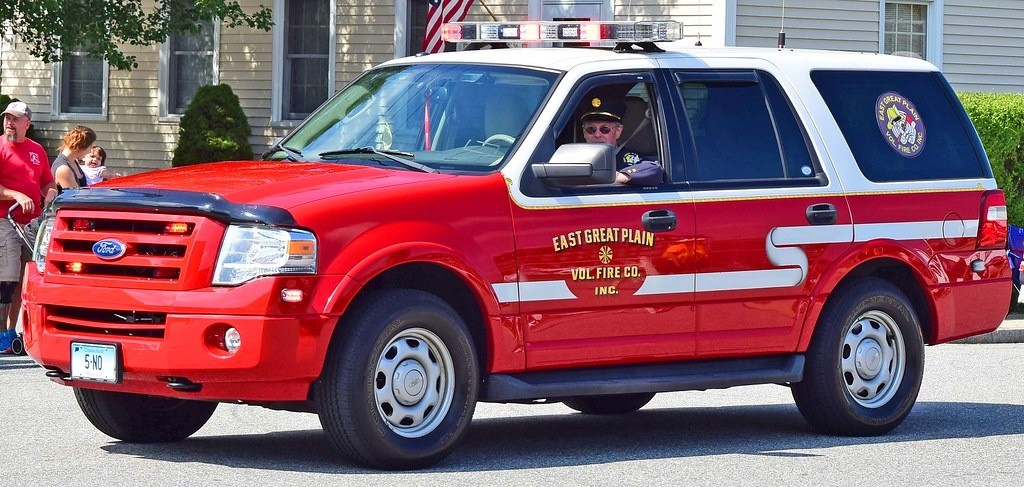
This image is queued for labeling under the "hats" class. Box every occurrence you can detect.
[0,102,32,120]
[574,96,628,123]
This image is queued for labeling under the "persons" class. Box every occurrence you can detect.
[50,126,97,189]
[580,95,664,186]
[0,102,58,352]
[79,146,121,185]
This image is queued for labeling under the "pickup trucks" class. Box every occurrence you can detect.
[22,22,1014,471]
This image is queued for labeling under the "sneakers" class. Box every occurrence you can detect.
[8,329,17,344]
[0,331,12,353]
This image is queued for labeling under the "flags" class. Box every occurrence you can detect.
[420,0,472,55]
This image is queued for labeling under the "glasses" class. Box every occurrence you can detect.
[581,125,619,134]
